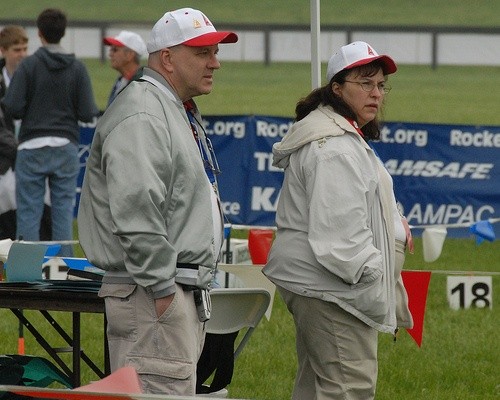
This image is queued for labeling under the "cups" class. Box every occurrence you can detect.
[0,239,12,282]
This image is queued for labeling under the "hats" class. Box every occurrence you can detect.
[104,30,146,57]
[147,8,239,53]
[327,41,397,82]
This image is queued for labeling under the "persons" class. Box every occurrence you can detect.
[261,41,416,400]
[0,8,97,257]
[104,30,147,109]
[0,23,53,242]
[78,8,239,397]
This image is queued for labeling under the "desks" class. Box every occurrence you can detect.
[0,281,108,385]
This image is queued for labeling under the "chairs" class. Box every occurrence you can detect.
[196,289,272,398]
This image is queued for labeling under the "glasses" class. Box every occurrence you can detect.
[343,78,392,96]
[109,47,119,52]
[198,138,222,175]
